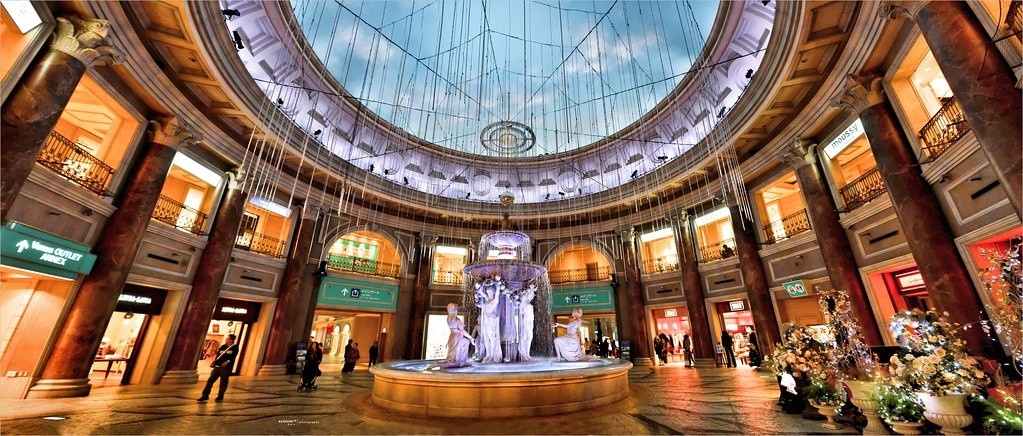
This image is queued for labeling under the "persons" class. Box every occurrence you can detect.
[653,333,675,366]
[341,339,360,373]
[552,308,596,361]
[304,342,322,390]
[720,245,735,258]
[427,303,476,370]
[721,331,736,368]
[478,282,502,363]
[584,337,616,358]
[683,335,694,366]
[196,334,239,401]
[735,332,747,365]
[369,341,378,367]
[521,287,534,360]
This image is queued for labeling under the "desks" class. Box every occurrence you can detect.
[94,355,128,378]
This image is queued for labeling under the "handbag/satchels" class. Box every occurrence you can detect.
[210,361,215,368]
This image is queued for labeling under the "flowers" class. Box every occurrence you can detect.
[764,285,1023,436]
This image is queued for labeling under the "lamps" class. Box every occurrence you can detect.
[228,311,236,327]
[124,311,133,319]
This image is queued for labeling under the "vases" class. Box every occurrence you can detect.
[838,378,889,436]
[808,399,842,429]
[915,391,973,436]
[885,418,923,436]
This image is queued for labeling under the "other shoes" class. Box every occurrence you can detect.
[216,395,223,401]
[197,395,208,401]
